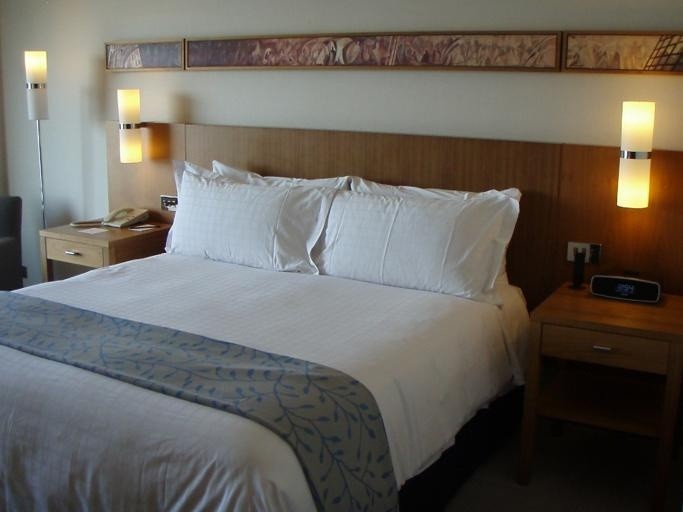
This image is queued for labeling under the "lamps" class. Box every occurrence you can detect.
[614,101,654,208]
[116,86,143,166]
[21,50,49,231]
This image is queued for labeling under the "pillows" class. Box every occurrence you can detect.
[308,188,520,305]
[161,159,348,279]
[350,172,522,287]
[207,160,350,197]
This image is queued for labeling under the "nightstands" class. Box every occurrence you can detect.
[520,277,682,509]
[36,215,173,283]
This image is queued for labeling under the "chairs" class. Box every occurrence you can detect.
[0,196,22,292]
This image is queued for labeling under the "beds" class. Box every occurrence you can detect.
[0,253,525,512]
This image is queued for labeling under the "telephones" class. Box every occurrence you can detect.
[99,207,149,229]
